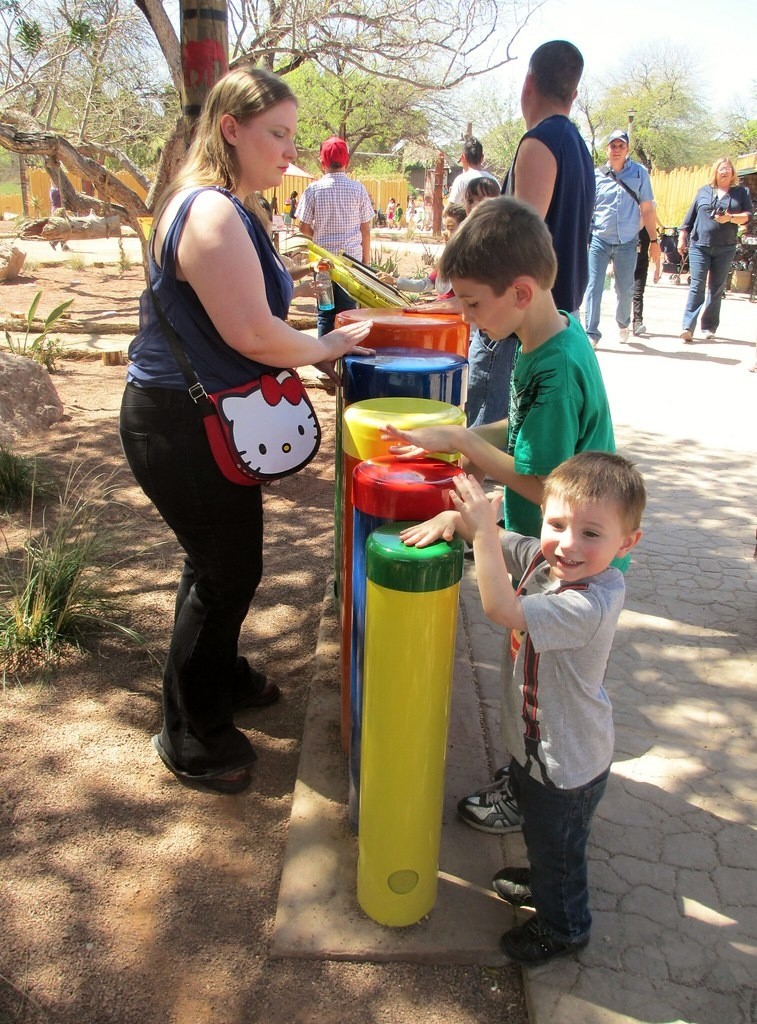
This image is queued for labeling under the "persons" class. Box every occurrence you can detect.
[50,179,74,253]
[678,158,753,343]
[399,450,648,965]
[463,41,597,427]
[379,195,632,834]
[255,137,500,379]
[585,130,662,348]
[120,65,374,793]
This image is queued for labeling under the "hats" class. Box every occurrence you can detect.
[285,198,290,205]
[320,137,347,168]
[607,129,628,145]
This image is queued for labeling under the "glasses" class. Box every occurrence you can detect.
[717,168,732,173]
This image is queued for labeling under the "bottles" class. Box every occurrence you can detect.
[315,265,335,310]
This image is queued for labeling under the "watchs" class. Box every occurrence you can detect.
[730,214,735,223]
[650,239,660,243]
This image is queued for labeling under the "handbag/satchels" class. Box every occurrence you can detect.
[197,368,322,485]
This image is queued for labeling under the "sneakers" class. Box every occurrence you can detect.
[501,913,589,964]
[457,766,522,834]
[492,866,536,907]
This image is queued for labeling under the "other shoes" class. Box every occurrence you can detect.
[680,330,692,341]
[317,370,330,379]
[239,678,280,710]
[620,327,629,340]
[706,331,715,339]
[198,767,252,794]
[587,336,597,351]
[633,322,646,335]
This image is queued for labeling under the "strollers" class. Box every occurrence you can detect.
[656,227,691,286]
